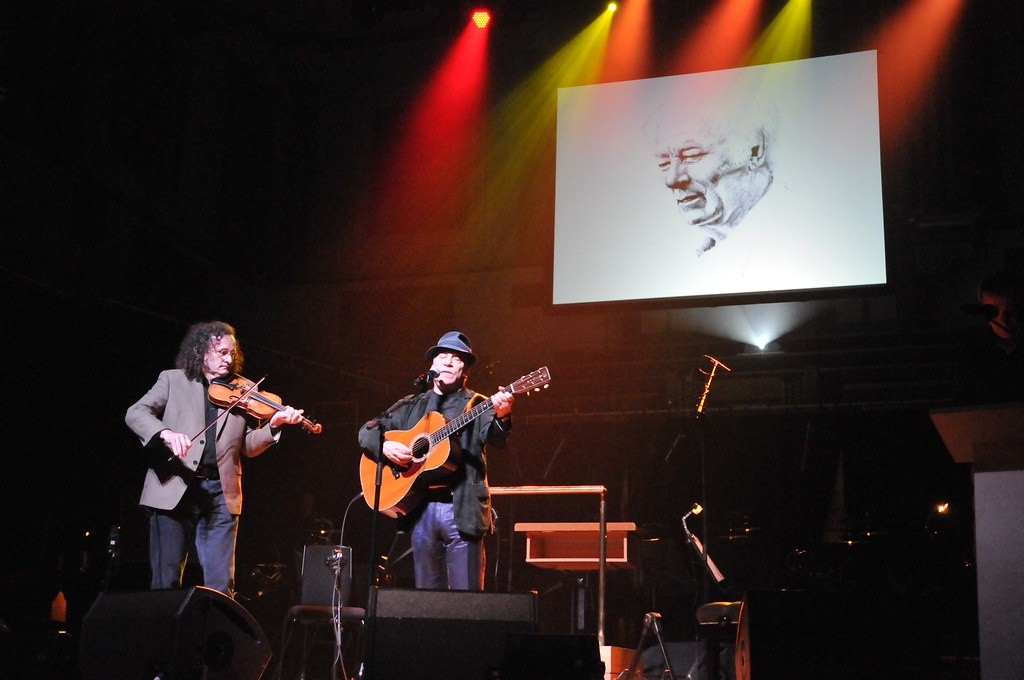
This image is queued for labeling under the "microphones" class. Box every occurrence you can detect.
[413,367,440,385]
[705,354,731,373]
[961,301,999,318]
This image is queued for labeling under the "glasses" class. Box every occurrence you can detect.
[212,350,237,356]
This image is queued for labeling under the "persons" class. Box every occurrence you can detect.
[125,322,305,597]
[357,331,515,591]
[640,97,876,295]
[932,270,1024,501]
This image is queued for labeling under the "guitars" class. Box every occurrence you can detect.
[360,364,552,519]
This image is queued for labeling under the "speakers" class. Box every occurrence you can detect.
[500,632,605,680]
[369,586,538,680]
[78,585,272,680]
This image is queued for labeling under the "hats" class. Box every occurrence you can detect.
[425,331,478,368]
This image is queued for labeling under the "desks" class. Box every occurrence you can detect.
[514,521,646,631]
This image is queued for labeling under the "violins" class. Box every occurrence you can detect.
[207,374,322,435]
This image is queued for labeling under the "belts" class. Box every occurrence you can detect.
[192,465,219,480]
